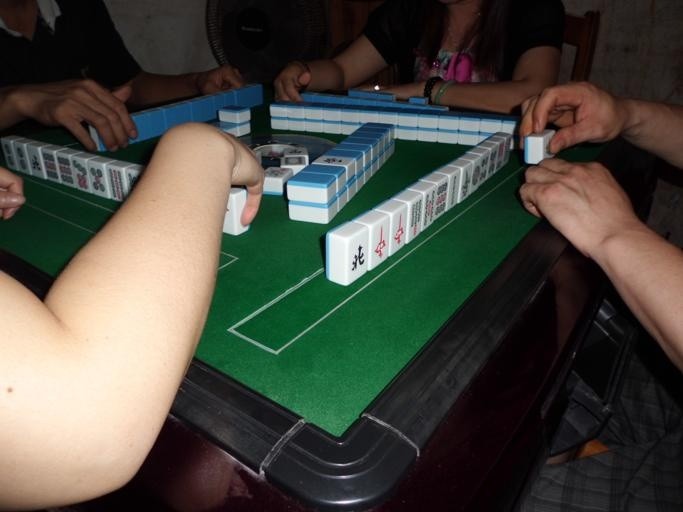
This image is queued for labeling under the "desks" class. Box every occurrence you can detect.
[0,87,615,512]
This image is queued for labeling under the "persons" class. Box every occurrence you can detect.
[0,0,249,155]
[515,79,682,512]
[0,122,266,511]
[274,1,567,116]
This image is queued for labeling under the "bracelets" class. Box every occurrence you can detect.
[423,75,443,106]
[434,78,458,107]
